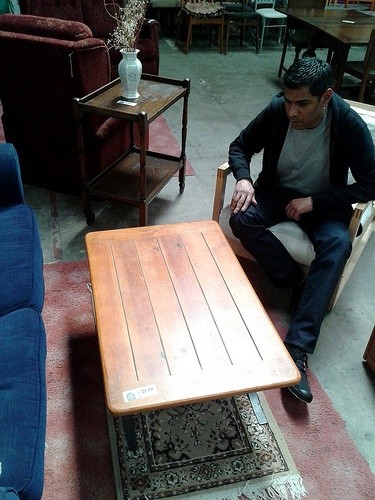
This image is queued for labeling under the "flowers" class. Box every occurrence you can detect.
[103,0,151,52]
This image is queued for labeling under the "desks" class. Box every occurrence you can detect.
[276,7,375,92]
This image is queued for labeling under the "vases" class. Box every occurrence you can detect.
[118,48,142,100]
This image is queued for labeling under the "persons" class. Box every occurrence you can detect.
[228,57,375,404]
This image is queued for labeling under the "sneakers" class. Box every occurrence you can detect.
[287,289,303,313]
[284,341,313,403]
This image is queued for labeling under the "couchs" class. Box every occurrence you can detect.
[0,142,47,500]
[0,0,162,195]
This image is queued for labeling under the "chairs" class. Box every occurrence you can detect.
[212,96,375,314]
[174,0,334,78]
[337,28,375,104]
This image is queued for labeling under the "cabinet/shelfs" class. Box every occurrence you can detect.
[74,69,190,227]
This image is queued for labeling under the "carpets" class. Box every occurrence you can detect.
[104,388,305,500]
[43,261,375,500]
[149,114,195,177]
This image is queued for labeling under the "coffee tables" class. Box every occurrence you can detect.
[85,220,300,449]
